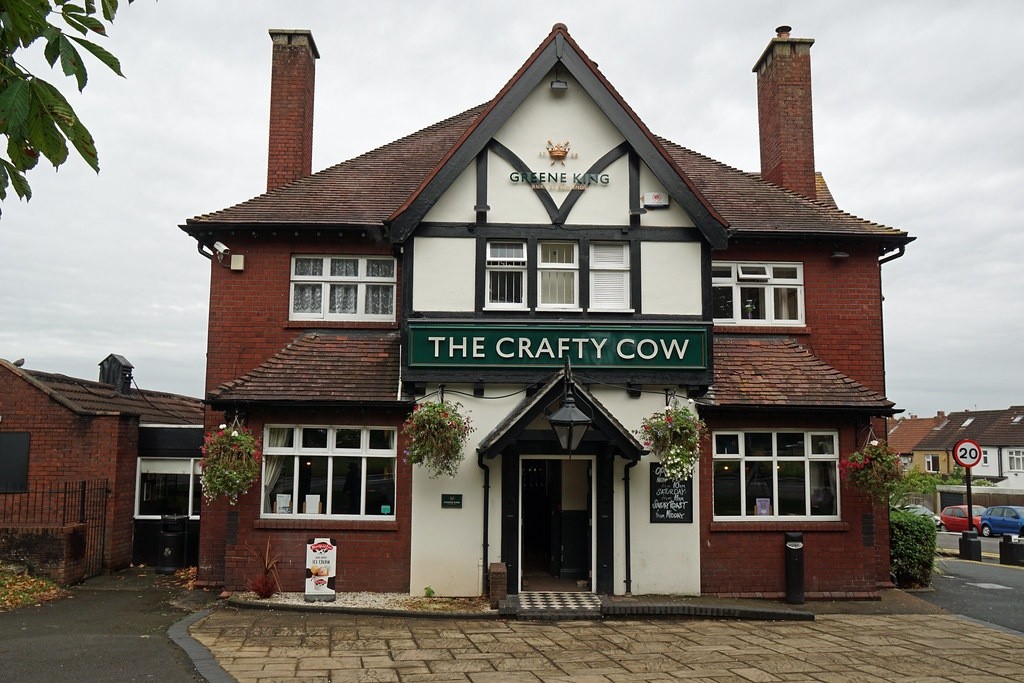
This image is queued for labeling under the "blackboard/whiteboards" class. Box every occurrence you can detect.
[649,461,694,524]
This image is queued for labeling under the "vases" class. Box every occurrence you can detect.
[748,311,752,319]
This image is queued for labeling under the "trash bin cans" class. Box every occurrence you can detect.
[784,530,804,605]
[156,515,186,575]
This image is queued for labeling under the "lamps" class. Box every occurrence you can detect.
[551,71,568,89]
[544,355,595,461]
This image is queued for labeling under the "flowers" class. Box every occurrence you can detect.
[838,438,904,502]
[631,398,710,483]
[745,299,756,313]
[400,400,477,479]
[197,420,263,506]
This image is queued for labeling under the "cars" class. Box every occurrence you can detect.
[981,506,1024,538]
[939,505,987,536]
[895,504,941,530]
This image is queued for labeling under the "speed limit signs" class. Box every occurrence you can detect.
[952,439,982,468]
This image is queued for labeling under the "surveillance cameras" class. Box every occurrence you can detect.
[213,241,231,268]
